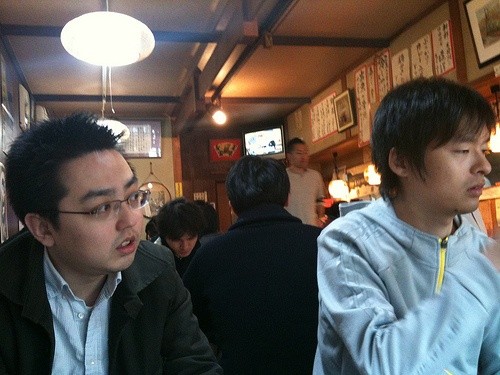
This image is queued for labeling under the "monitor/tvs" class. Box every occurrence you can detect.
[242,125,286,160]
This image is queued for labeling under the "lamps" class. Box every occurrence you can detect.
[58,0,157,145]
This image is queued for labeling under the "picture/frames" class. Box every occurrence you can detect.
[332,87,358,134]
[460,0,500,70]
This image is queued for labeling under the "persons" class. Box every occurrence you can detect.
[180,155,326,375]
[313,75,500,375]
[0,113,223,375]
[142,137,326,278]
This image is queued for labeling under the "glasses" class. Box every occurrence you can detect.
[53,190,151,222]
